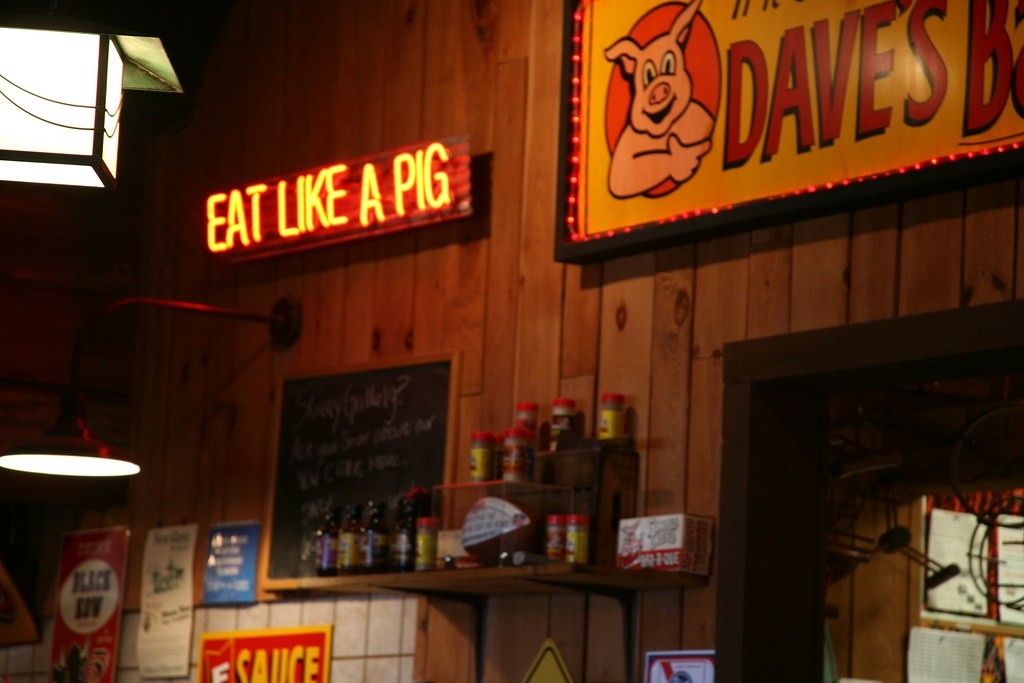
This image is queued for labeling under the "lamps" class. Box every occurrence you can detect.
[0,294,304,478]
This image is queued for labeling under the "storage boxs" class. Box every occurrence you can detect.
[615,512,713,577]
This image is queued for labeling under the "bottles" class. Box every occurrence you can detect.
[503,428,527,482]
[359,502,388,573]
[315,505,338,575]
[515,402,539,481]
[416,517,440,571]
[469,431,489,482]
[546,514,566,559]
[335,505,359,573]
[389,501,417,572]
[489,433,504,479]
[551,398,576,430]
[566,514,589,562]
[597,392,625,439]
